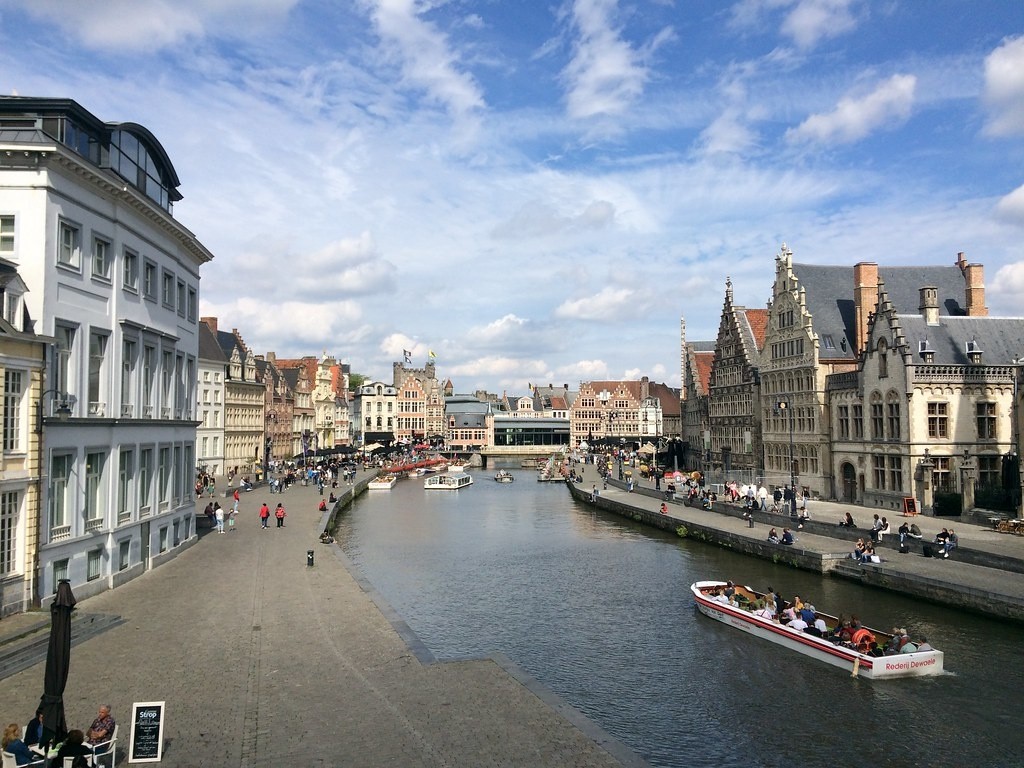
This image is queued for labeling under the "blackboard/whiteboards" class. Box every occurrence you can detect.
[128,701,166,763]
[904,498,917,514]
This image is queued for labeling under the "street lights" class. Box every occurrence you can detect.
[609,408,623,480]
[773,393,798,516]
[642,403,660,490]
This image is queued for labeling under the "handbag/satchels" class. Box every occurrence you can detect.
[870,555,881,564]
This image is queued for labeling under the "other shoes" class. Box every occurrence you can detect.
[944,554,949,557]
[900,542,904,547]
[261,525,264,529]
[938,549,945,553]
[281,526,286,528]
[264,527,267,529]
[798,523,803,528]
[749,515,751,518]
[218,532,220,534]
[277,527,279,529]
[743,513,747,516]
[877,540,882,544]
[221,532,225,533]
[98,763,105,768]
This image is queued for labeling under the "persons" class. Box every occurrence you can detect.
[469,443,472,451]
[269,462,357,511]
[666,483,675,500]
[195,472,253,534]
[364,452,430,468]
[500,468,504,474]
[23,707,43,745]
[2,723,34,765]
[768,527,794,545]
[712,581,932,657]
[798,507,811,528]
[482,443,484,449]
[568,456,654,502]
[56,730,96,768]
[259,503,270,530]
[802,487,810,507]
[439,443,443,451]
[842,512,957,567]
[681,474,717,510]
[660,502,668,513]
[275,503,287,529]
[86,704,115,768]
[725,481,791,518]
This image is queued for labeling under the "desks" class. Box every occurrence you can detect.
[988,518,1001,531]
[28,739,65,760]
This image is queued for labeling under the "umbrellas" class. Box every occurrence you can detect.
[39,579,77,768]
[295,447,355,457]
[430,434,445,439]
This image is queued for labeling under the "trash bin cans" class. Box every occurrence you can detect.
[307,549,315,566]
[255,470,263,481]
[923,545,933,558]
[748,517,755,528]
[781,504,790,514]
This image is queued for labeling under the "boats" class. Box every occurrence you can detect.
[424,470,474,490]
[494,472,514,483]
[367,474,398,489]
[690,579,946,681]
[406,459,473,479]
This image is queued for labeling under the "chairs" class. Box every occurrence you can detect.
[1,747,45,768]
[63,754,92,768]
[20,726,27,742]
[83,724,119,768]
